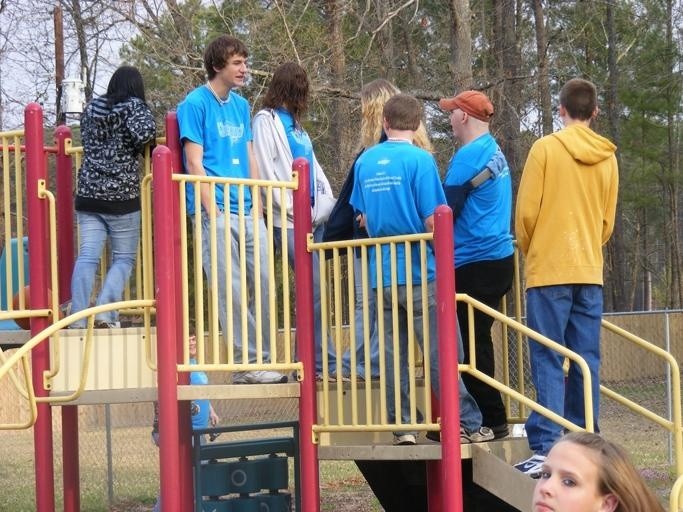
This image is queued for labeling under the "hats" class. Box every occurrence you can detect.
[439,90,494,122]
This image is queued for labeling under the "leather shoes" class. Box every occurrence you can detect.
[490,422,509,439]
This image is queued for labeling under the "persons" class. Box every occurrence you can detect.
[251,60,356,384]
[531,429,665,511]
[431,90,518,440]
[320,78,434,385]
[66,66,157,328]
[513,77,620,481]
[153,323,220,512]
[346,93,497,447]
[175,35,289,385]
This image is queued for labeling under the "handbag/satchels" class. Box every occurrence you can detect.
[441,182,469,222]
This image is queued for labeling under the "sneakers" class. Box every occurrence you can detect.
[232,366,289,384]
[460,424,496,444]
[393,434,417,445]
[511,453,547,477]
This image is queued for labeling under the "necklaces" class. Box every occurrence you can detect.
[388,138,413,144]
[206,81,231,106]
